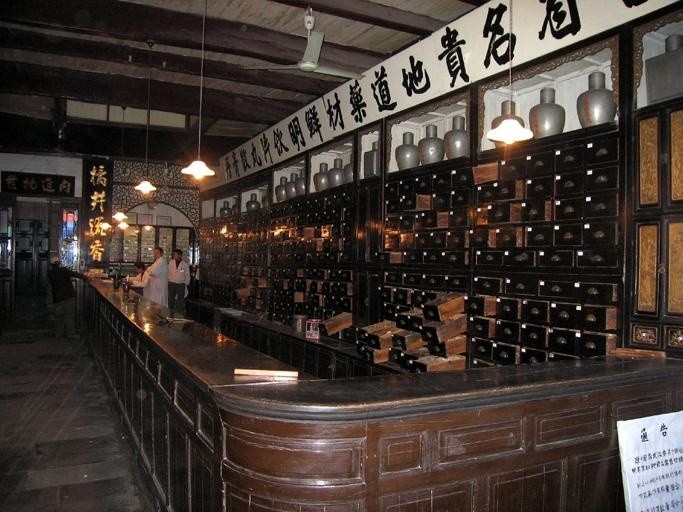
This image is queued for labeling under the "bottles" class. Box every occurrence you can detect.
[245,189,269,211]
[576,71,616,128]
[394,115,467,171]
[313,155,352,191]
[490,100,524,148]
[363,142,379,179]
[113,273,119,290]
[528,88,565,139]
[293,315,307,336]
[274,169,305,203]
[220,195,237,218]
[664,34,682,51]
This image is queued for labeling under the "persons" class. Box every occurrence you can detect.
[47,255,88,338]
[168,248,192,317]
[144,247,169,307]
[126,262,153,288]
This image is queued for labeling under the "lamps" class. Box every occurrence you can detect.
[132,38,161,197]
[178,1,218,181]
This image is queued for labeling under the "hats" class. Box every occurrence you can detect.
[50,256,60,264]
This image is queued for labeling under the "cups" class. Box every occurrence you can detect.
[123,284,129,293]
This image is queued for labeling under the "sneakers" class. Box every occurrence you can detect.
[68,334,81,339]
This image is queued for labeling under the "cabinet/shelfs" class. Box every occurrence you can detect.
[197,0,682,380]
